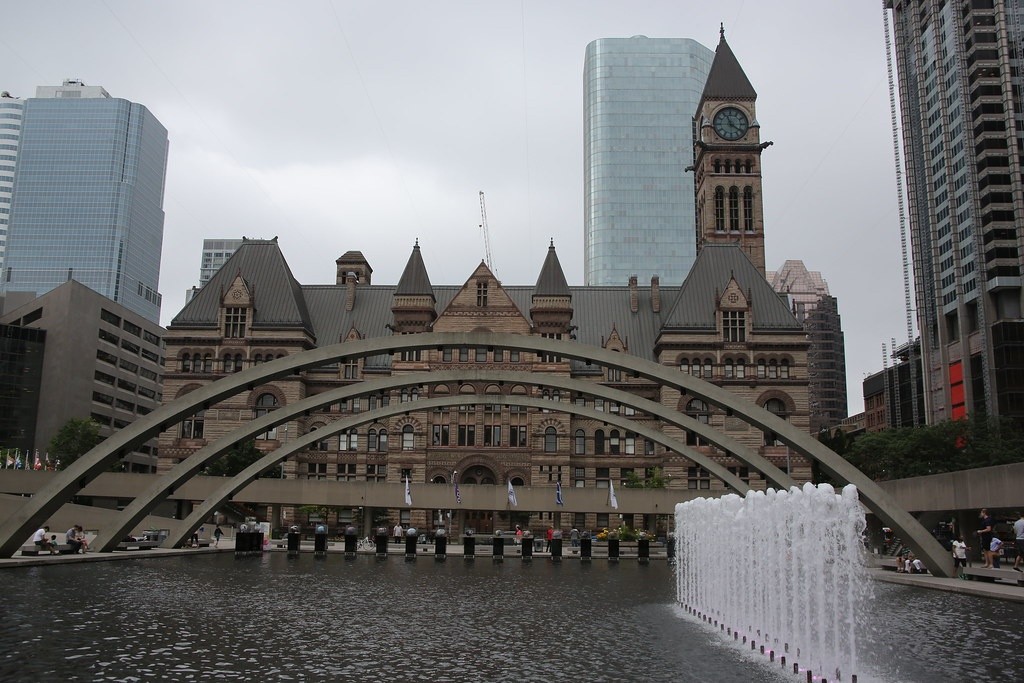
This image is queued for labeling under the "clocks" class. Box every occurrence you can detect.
[713,107,749,141]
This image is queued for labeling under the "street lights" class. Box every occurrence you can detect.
[479,190,500,282]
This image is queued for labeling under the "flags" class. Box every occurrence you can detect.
[7,451,60,470]
[610,482,618,510]
[556,482,564,505]
[405,478,412,505]
[454,481,462,504]
[508,481,517,506]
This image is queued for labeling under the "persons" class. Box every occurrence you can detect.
[513,525,522,546]
[884,528,893,549]
[570,527,590,553]
[34,526,59,554]
[393,522,404,544]
[952,533,972,578]
[214,525,224,547]
[977,509,1004,580]
[65,525,90,554]
[183,531,200,549]
[1014,513,1024,571]
[546,526,562,552]
[419,528,435,544]
[896,542,923,573]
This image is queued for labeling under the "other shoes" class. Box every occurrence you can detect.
[197,546,200,548]
[74,551,82,554]
[214,544,218,548]
[988,566,994,570]
[54,552,63,555]
[981,565,988,568]
[1013,566,1023,572]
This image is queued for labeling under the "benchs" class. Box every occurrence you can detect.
[876,558,899,570]
[963,566,1024,585]
[183,539,215,547]
[117,541,159,550]
[17,543,78,555]
